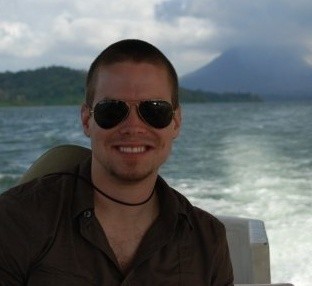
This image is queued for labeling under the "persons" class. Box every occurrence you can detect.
[0,40,234,286]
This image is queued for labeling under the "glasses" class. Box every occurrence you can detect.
[88,100,175,128]
